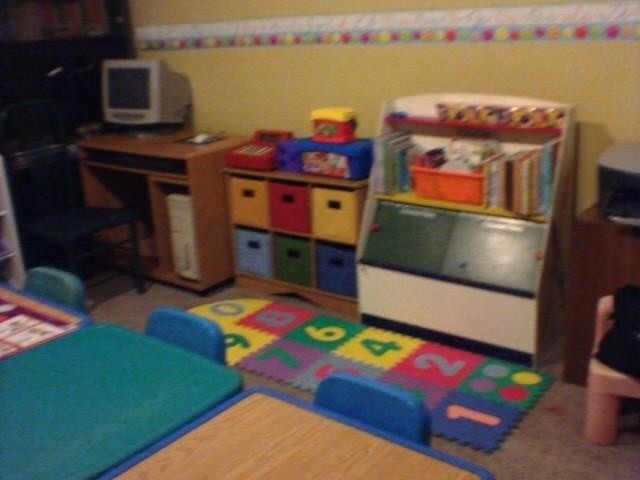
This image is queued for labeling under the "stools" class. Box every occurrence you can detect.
[579,293,640,448]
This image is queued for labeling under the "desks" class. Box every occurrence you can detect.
[1,322,245,480]
[79,129,251,297]
[93,383,496,480]
[1,287,90,363]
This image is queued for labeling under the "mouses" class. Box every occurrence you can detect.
[192,134,213,145]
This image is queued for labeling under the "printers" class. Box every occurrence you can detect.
[594,139,640,230]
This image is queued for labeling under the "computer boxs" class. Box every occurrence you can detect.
[165,194,198,281]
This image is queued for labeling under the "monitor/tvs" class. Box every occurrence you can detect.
[101,58,189,137]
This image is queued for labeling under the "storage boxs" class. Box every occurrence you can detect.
[407,157,485,207]
[317,245,354,297]
[311,185,368,244]
[269,180,311,235]
[272,236,310,287]
[226,175,268,231]
[308,104,360,145]
[294,134,372,182]
[235,228,273,280]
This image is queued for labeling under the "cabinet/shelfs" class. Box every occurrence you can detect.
[559,199,639,395]
[356,88,581,370]
[222,165,369,317]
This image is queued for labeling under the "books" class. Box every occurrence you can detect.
[370,131,562,217]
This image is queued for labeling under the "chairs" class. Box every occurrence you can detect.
[308,372,432,446]
[145,307,227,364]
[18,265,85,312]
[12,161,142,297]
